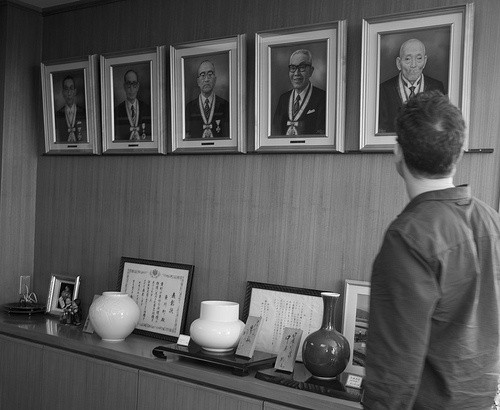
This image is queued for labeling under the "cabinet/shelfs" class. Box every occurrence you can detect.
[0,324,361,410]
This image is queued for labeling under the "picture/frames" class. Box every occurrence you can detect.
[254,20,346,154]
[361,2,469,153]
[169,33,249,155]
[99,44,167,155]
[340,279,374,375]
[40,53,99,157]
[46,275,81,317]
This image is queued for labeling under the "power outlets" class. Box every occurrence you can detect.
[19,275,31,295]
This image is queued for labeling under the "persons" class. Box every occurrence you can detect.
[185,59,229,138]
[363,89,500,410]
[378,39,447,133]
[59,286,72,306]
[62,298,81,326]
[114,70,152,140]
[274,49,326,135]
[55,76,87,143]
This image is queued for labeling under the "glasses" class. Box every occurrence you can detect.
[125,80,139,88]
[288,63,310,72]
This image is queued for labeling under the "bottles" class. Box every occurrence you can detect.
[302,292,351,380]
[190,300,246,353]
[88,291,140,341]
